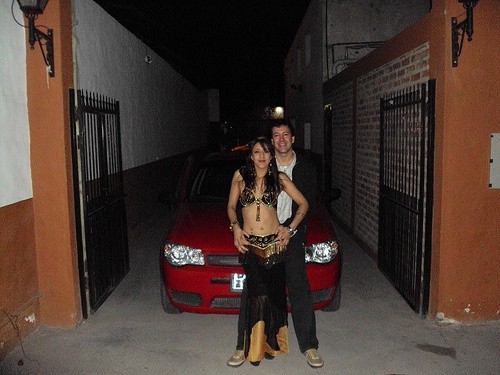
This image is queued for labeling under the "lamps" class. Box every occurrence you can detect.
[10,0,56,81]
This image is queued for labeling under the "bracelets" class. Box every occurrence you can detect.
[286,225,294,235]
[229,220,239,232]
[295,212,305,217]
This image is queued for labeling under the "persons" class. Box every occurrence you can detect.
[227,138,307,366]
[228,117,324,369]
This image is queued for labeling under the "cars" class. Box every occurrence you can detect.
[158,144,343,314]
